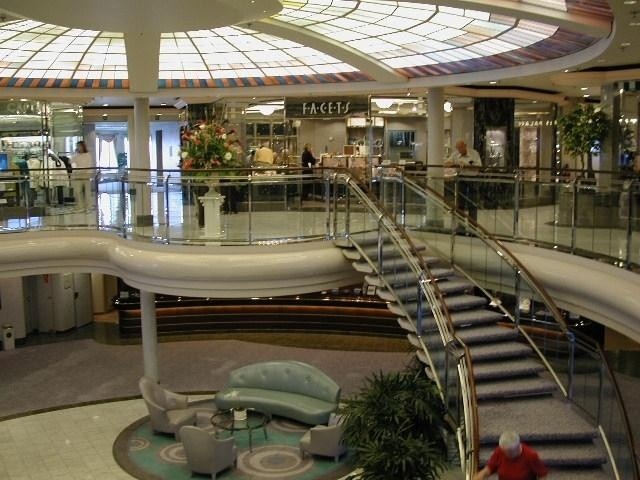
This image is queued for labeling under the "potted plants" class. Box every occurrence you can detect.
[554,104,621,229]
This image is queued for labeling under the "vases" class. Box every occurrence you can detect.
[198,185,227,247]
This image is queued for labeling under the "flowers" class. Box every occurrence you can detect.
[178,101,245,185]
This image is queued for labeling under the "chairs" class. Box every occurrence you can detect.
[138,377,197,442]
[179,425,238,480]
[299,412,349,464]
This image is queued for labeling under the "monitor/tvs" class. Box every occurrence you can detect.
[0,151,9,173]
[590,141,600,153]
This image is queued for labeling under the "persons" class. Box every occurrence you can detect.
[67,140,95,215]
[246,150,257,174]
[470,429,551,480]
[302,142,320,205]
[257,145,274,174]
[224,128,246,215]
[443,138,483,236]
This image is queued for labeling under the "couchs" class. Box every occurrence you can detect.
[215,359,342,425]
